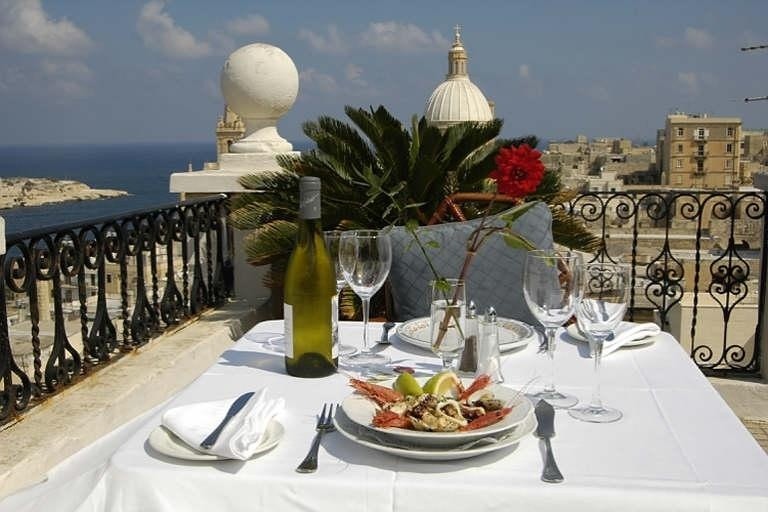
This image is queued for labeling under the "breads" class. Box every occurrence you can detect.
[401,388,507,431]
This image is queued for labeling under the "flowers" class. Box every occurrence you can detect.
[433,142,546,349]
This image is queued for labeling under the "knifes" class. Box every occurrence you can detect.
[535,396,563,483]
[201,390,254,450]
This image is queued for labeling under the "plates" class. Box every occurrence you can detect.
[332,315,539,462]
[147,425,287,462]
[568,321,655,347]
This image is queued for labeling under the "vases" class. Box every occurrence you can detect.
[430,277,468,368]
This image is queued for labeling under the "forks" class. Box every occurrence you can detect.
[298,402,339,473]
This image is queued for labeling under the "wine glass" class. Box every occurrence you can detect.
[429,281,464,373]
[323,230,392,366]
[521,249,632,423]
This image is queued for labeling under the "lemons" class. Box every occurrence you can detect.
[394,372,425,397]
[422,370,464,398]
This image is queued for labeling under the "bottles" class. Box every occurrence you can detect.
[463,301,497,382]
[281,174,339,378]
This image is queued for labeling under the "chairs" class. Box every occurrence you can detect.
[385,191,574,327]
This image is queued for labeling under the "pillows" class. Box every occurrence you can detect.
[376,200,562,331]
